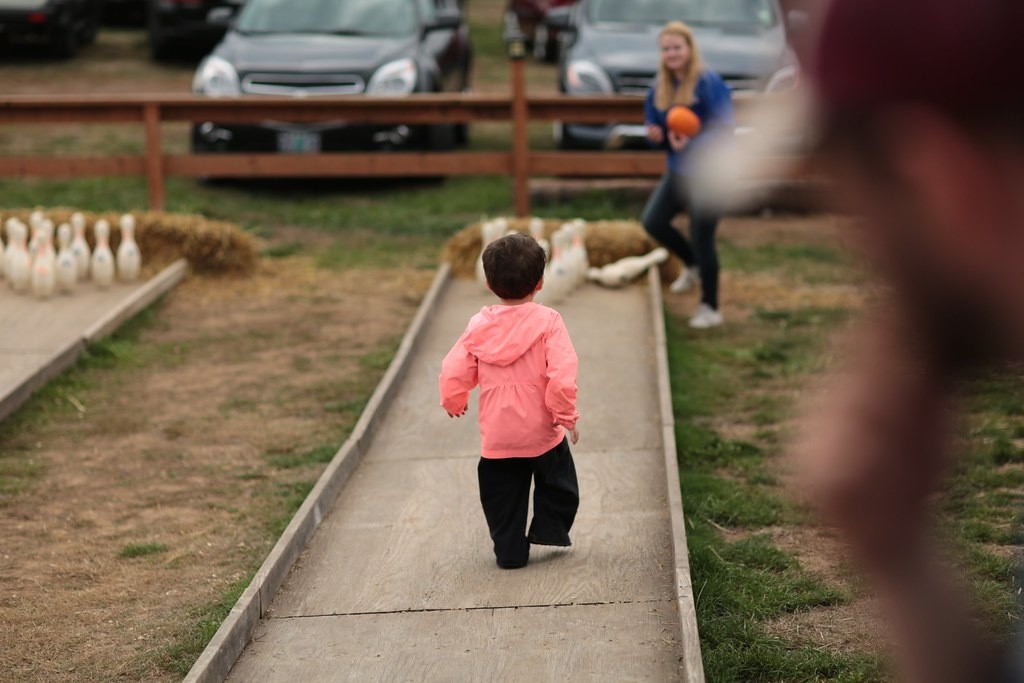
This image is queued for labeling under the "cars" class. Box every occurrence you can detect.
[549,1,802,177]
[0,0,241,67]
[188,0,475,193]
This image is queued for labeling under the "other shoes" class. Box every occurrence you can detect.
[528,533,572,546]
[670,266,699,293]
[689,303,724,328]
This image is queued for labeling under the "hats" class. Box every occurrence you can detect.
[690,1,1024,217]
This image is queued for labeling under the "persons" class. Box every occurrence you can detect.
[438,234,581,568]
[689,0,1024,683]
[641,20,734,329]
